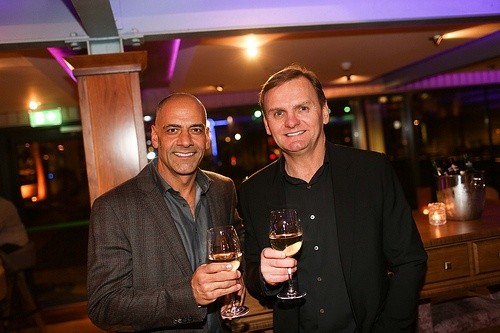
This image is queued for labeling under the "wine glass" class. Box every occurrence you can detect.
[269,209,307,299]
[208,225,249,319]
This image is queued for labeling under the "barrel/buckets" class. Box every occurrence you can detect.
[436,175,485,220]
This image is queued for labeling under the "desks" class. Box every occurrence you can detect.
[229,186,500,333]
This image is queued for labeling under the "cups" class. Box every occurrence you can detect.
[428,202,446,225]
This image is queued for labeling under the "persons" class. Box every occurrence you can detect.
[239,64,428,333]
[86,93,245,333]
[0,197,30,254]
